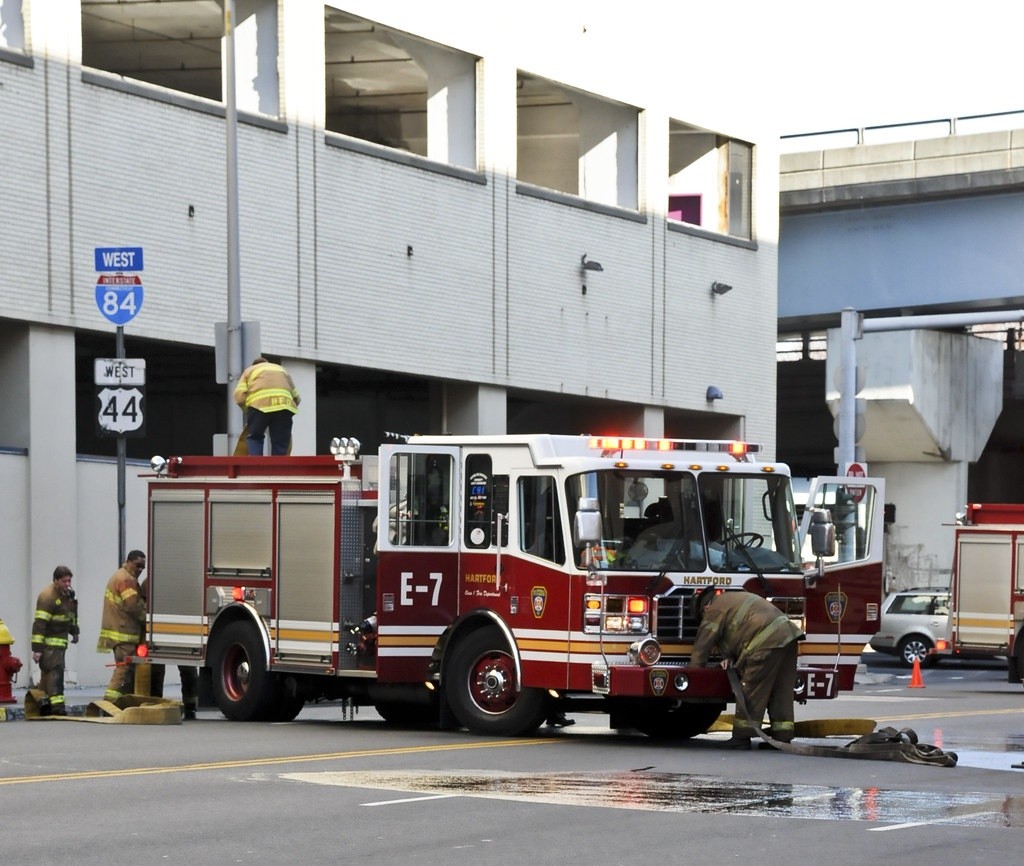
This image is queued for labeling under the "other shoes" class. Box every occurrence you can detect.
[716,737,751,751]
[185,710,196,720]
[757,740,791,750]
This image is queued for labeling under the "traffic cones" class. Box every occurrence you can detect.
[908,658,926,688]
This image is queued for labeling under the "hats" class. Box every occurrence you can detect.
[689,585,716,618]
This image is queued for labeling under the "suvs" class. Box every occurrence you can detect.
[868,587,952,668]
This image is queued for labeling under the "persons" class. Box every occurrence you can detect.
[31,565,80,715]
[140,574,200,721]
[233,357,301,456]
[96,550,148,717]
[690,584,806,750]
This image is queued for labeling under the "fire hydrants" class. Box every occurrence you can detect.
[0,620,23,703]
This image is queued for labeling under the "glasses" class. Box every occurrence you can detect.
[132,561,146,569]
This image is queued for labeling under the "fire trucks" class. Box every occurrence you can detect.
[942,501,1024,684]
[107,431,886,742]
